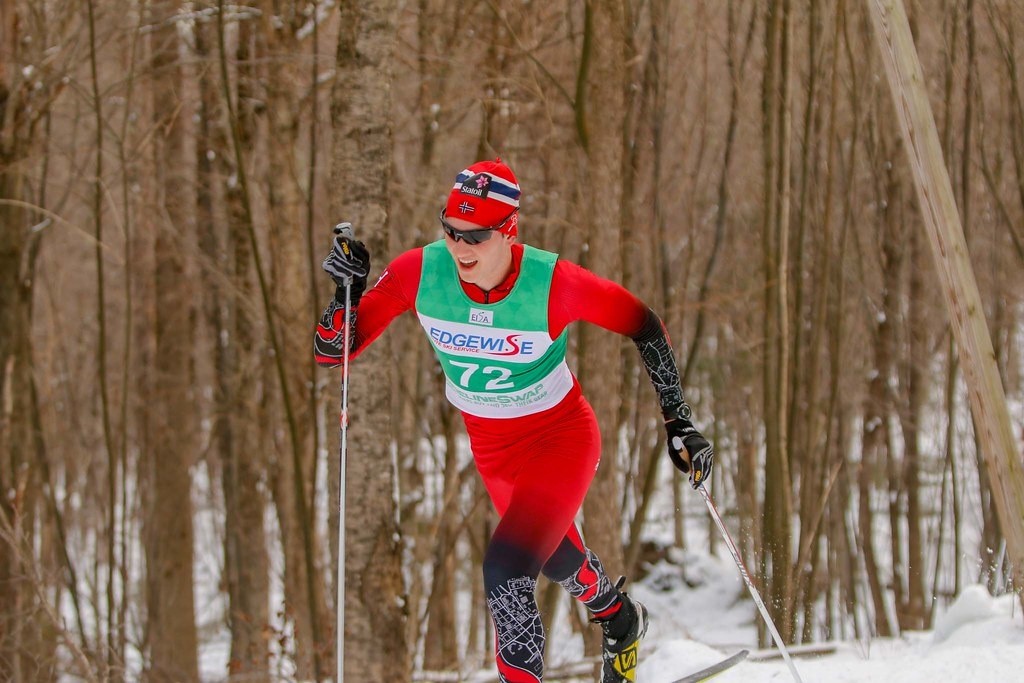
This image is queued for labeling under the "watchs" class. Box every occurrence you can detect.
[663,402,692,421]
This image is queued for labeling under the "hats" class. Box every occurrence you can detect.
[446,157,520,237]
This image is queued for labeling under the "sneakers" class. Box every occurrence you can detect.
[591,601,649,683]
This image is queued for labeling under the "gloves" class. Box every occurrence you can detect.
[662,412,713,490]
[322,227,371,304]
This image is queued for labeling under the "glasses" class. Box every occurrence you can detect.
[440,208,520,245]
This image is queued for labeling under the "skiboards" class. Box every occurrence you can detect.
[671,647,751,683]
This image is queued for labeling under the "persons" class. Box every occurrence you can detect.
[313,158,713,683]
